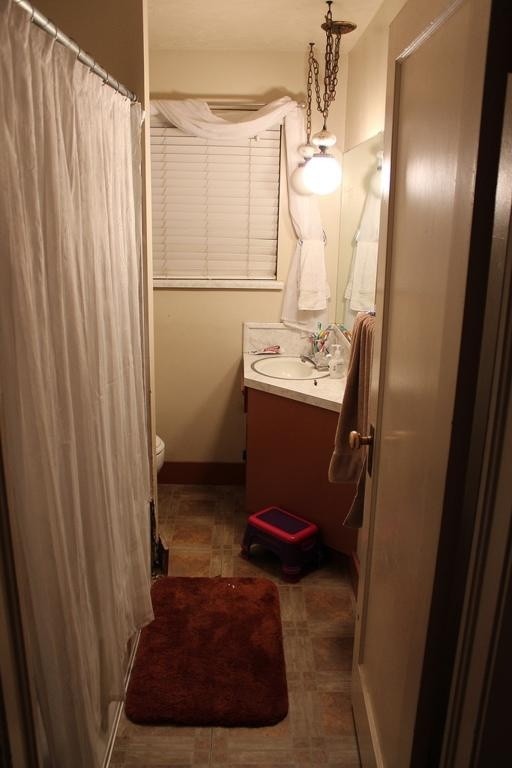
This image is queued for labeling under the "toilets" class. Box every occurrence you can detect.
[156,434,166,474]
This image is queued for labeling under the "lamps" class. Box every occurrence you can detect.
[291,0,353,194]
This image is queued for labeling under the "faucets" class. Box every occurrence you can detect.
[300,354,331,371]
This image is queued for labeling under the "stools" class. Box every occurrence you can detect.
[239,506,323,584]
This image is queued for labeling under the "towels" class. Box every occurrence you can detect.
[326,312,377,528]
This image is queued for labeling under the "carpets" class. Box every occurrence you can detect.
[125,576,288,727]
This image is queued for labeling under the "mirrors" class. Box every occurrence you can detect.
[335,132,382,343]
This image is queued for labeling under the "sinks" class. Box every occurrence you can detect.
[251,356,331,380]
